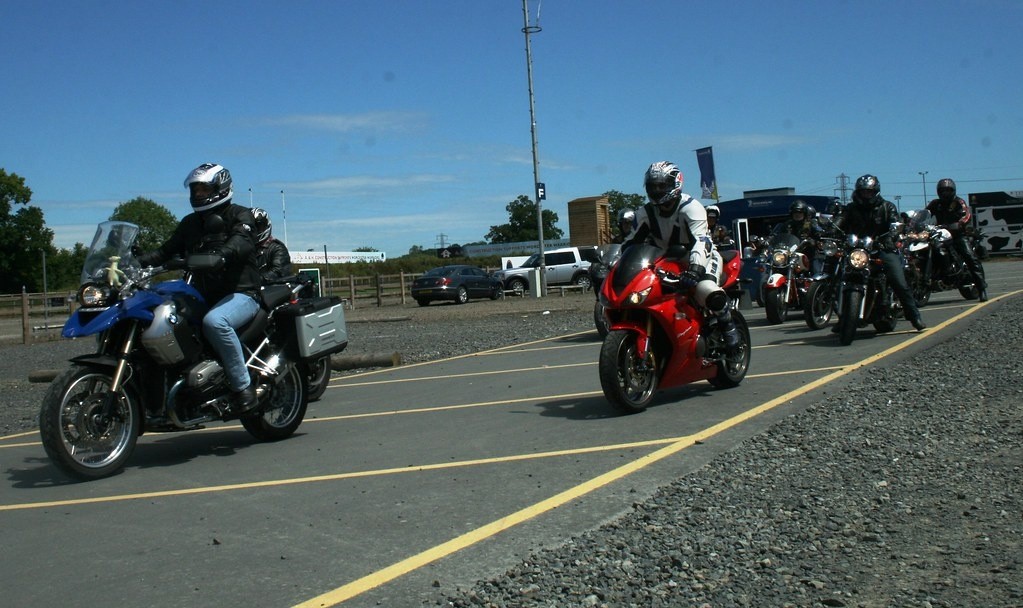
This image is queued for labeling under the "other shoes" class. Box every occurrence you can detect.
[912,320,926,331]
[831,322,841,333]
[979,290,987,301]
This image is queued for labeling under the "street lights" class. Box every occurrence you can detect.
[894,195,901,211]
[918,171,929,207]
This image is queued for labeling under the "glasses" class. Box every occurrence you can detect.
[192,185,210,192]
[650,183,667,190]
[860,189,872,192]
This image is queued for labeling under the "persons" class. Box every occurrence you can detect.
[703,205,735,245]
[611,207,635,243]
[619,161,740,351]
[747,172,989,333]
[96,165,263,413]
[251,204,294,282]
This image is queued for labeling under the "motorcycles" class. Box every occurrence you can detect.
[597,208,751,415]
[589,243,623,340]
[38,221,349,481]
[739,197,988,346]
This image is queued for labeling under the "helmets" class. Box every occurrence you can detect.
[645,161,683,206]
[250,208,272,246]
[937,179,956,200]
[717,225,727,235]
[616,208,635,230]
[806,205,816,218]
[791,200,807,218]
[749,234,761,245]
[706,206,720,220]
[184,164,233,216]
[856,175,879,204]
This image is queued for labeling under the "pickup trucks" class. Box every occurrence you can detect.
[492,246,592,297]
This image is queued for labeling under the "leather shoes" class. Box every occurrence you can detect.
[235,382,259,413]
[727,352,743,376]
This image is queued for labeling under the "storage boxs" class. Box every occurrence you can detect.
[272,296,348,364]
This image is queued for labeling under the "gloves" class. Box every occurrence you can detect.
[949,222,960,233]
[187,254,216,270]
[679,264,706,291]
[885,236,894,252]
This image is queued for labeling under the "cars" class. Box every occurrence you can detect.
[411,265,504,307]
